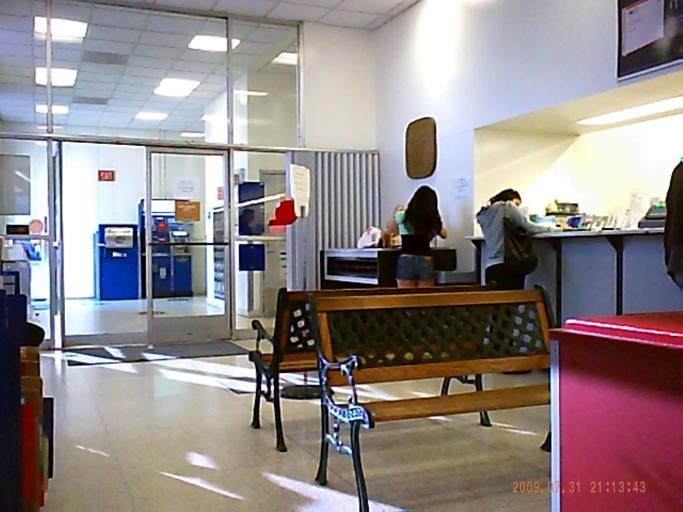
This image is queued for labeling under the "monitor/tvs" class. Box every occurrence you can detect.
[614,0,683,84]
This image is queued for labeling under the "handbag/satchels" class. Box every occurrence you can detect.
[503,216,537,274]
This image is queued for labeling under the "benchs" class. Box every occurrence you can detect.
[248,284,492,453]
[305,286,557,512]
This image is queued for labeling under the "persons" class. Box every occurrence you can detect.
[476,188,565,375]
[239,209,256,271]
[386,185,449,292]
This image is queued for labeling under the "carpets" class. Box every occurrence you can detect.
[62,338,249,367]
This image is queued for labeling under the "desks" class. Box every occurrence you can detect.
[320,248,456,289]
